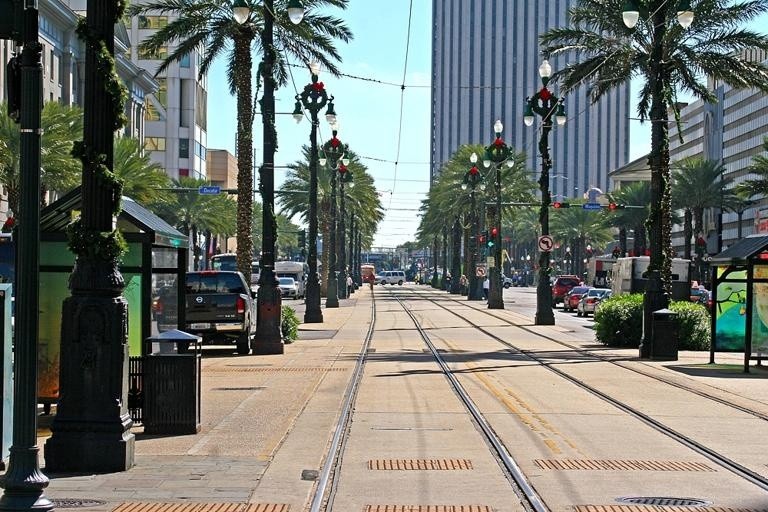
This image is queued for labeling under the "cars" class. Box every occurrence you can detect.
[690,287,712,313]
[152,279,172,320]
[551,274,612,316]
[414,270,443,284]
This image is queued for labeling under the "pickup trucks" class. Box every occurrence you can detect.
[155,271,256,354]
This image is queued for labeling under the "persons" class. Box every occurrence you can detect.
[346,273,354,297]
[482,275,490,304]
[464,275,470,296]
[459,274,467,296]
[444,272,451,292]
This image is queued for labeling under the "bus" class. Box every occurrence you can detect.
[360,262,375,282]
[210,253,237,271]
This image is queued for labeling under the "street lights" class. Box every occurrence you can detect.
[619,1,694,360]
[293,57,359,322]
[230,0,305,355]
[461,59,568,326]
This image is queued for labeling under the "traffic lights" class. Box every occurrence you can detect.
[697,236,707,250]
[553,201,561,210]
[608,203,626,210]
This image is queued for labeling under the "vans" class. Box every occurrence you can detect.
[375,271,406,285]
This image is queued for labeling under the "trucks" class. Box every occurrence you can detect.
[251,261,308,299]
[587,256,692,306]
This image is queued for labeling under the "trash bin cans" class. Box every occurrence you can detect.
[649,308,678,361]
[142,328,202,435]
